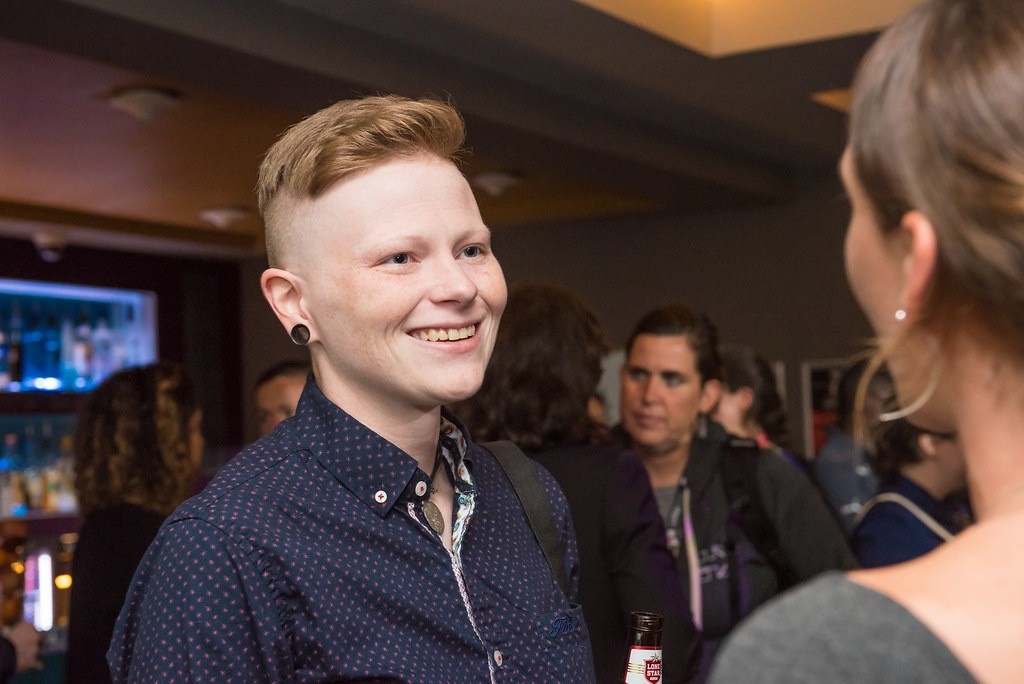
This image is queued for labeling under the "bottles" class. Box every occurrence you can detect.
[614,611,667,684]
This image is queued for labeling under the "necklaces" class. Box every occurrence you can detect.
[422,440,445,535]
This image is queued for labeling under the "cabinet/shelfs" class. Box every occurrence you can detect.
[0,276,156,659]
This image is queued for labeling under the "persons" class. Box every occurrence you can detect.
[250,362,309,436]
[105,98,594,684]
[0,547,44,684]
[708,1,1024,684]
[445,282,979,684]
[65,364,211,683]
